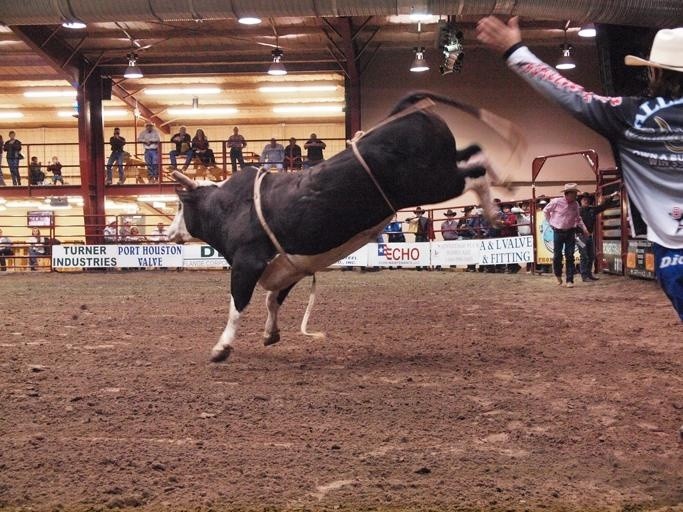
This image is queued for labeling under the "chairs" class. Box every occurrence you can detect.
[43,238,49,255]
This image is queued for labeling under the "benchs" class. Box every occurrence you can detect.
[103,152,307,183]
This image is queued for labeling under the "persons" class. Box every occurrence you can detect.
[384,184,614,288]
[107,127,126,184]
[192,129,215,167]
[260,138,284,171]
[138,124,160,179]
[0,229,8,271]
[28,227,45,271]
[170,127,193,171]
[304,133,326,160]
[104,221,168,244]
[476,14,683,326]
[227,127,247,173]
[284,138,302,169]
[0,131,65,188]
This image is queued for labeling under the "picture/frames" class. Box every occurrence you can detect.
[27,209,54,228]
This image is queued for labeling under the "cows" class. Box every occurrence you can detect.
[167,89,526,363]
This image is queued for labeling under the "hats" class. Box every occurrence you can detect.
[414,206,424,213]
[624,28,683,72]
[577,191,594,201]
[444,206,474,215]
[561,183,580,193]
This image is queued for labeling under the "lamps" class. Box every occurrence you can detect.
[124,38,143,78]
[62,22,88,29]
[556,27,575,70]
[237,17,262,25]
[578,23,597,38]
[410,20,430,72]
[267,18,287,75]
